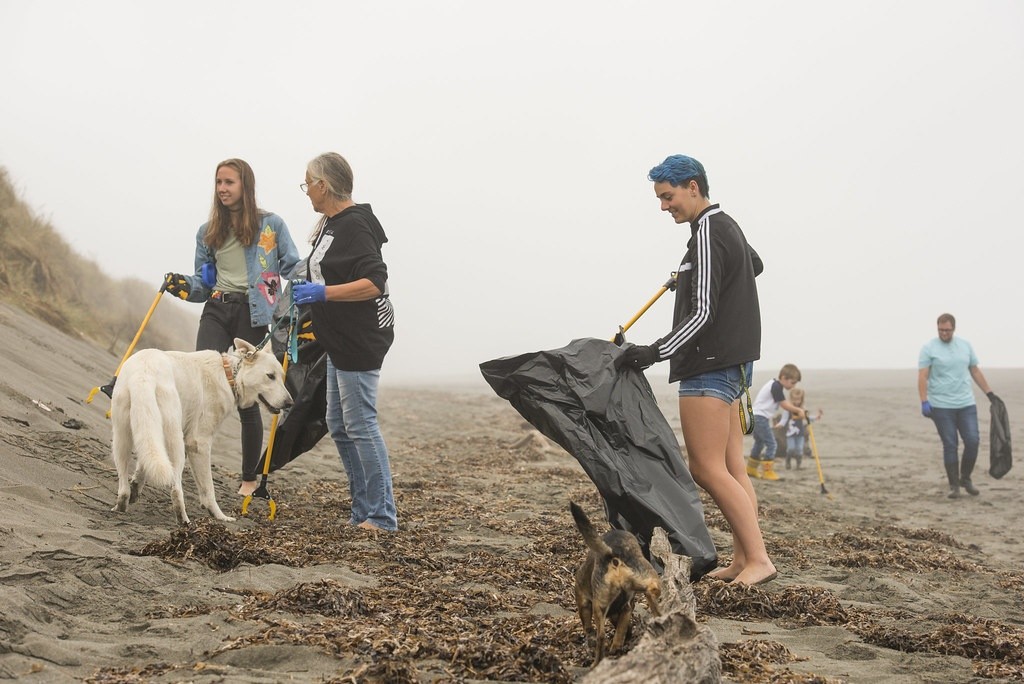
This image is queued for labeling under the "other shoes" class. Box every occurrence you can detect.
[785,460,791,469]
[796,466,804,470]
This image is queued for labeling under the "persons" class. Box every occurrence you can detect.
[745,364,805,481]
[607,155,777,590]
[165,158,300,497]
[290,152,398,533]
[773,388,824,469]
[918,313,996,498]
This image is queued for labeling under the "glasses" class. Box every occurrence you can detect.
[300,181,313,192]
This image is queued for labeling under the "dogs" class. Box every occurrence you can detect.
[110,336,294,527]
[569,500,662,668]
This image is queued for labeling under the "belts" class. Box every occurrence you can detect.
[209,291,249,304]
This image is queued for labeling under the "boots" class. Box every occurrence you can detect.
[960,467,980,495]
[746,456,761,477]
[761,460,779,479]
[947,470,961,498]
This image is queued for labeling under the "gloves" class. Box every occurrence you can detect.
[922,400,933,417]
[166,273,191,300]
[986,391,1002,404]
[292,279,325,304]
[614,343,660,372]
[670,273,678,291]
